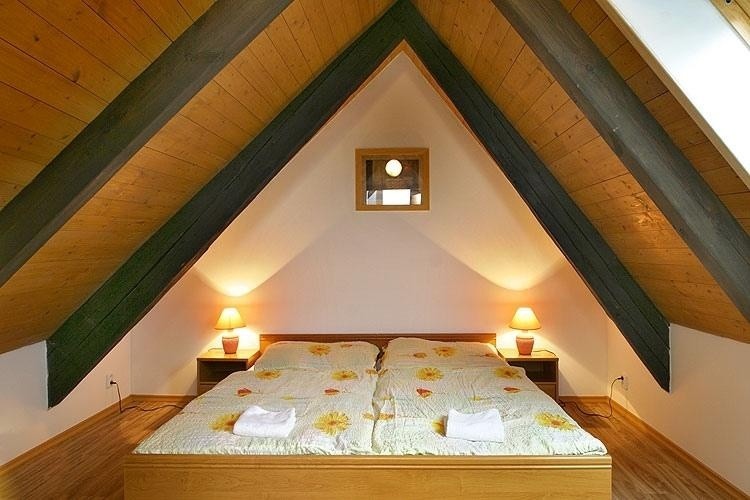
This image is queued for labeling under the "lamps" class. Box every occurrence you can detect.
[213,306,246,355]
[509,306,542,355]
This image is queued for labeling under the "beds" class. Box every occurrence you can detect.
[124,334,611,499]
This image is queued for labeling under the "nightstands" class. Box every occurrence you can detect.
[497,348,558,406]
[196,347,260,396]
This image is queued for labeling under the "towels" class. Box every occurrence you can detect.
[232,405,297,437]
[443,407,506,444]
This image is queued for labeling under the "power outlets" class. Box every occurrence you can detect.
[621,374,629,391]
[105,378,114,390]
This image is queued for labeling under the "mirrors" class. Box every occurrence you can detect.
[354,148,430,211]
